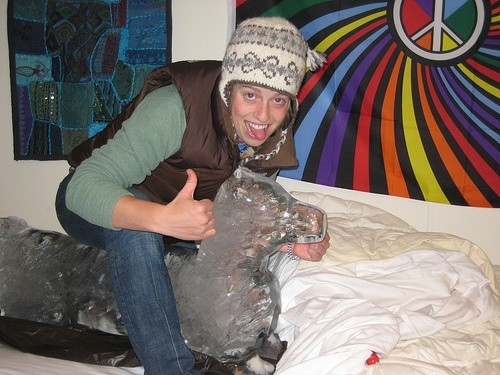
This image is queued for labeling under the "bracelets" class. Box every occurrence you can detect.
[285,239,302,261]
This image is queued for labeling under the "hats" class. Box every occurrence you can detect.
[218,15,328,179]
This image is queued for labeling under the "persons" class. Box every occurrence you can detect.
[53,15,331,375]
